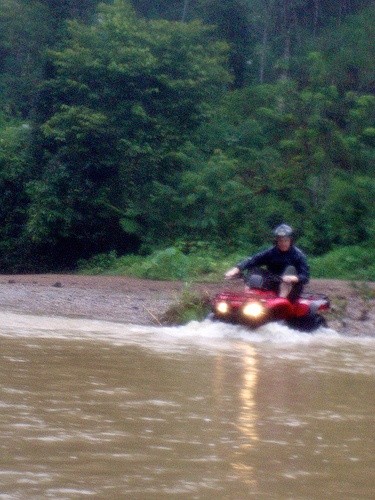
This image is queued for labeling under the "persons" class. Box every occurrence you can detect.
[225,225,310,302]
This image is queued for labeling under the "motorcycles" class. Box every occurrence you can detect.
[211,270,331,337]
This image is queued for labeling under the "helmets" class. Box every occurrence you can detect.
[273,224,295,244]
